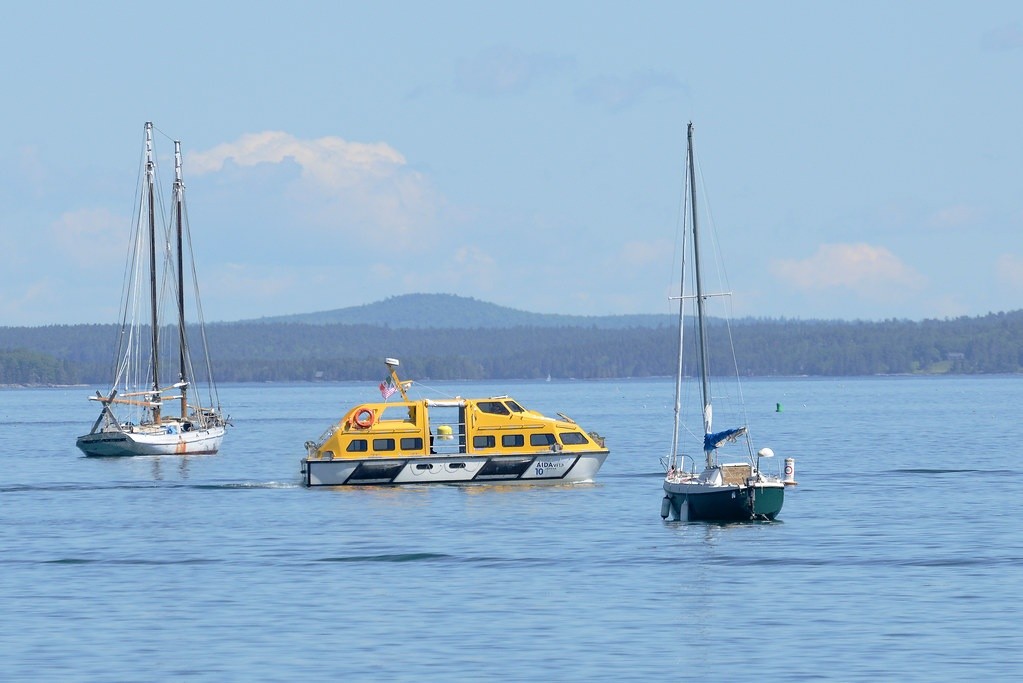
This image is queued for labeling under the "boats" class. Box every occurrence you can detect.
[299,356,612,486]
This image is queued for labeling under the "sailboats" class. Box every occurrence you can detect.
[655,118,800,526]
[75,120,236,458]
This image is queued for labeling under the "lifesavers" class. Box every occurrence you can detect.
[354,408,375,428]
